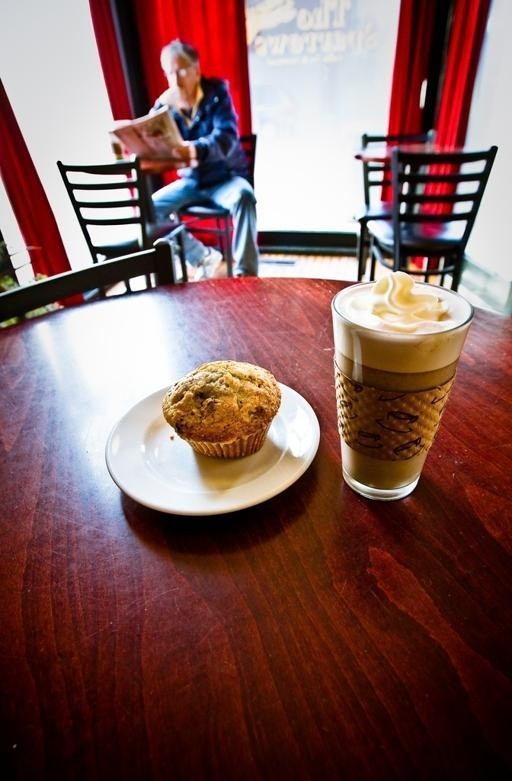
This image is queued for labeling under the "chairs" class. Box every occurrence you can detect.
[0,238,180,325]
[353,132,441,281]
[367,145,498,293]
[56,155,188,295]
[173,136,264,278]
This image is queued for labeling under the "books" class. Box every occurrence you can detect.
[108,105,184,159]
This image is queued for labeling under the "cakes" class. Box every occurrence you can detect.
[159,359,283,461]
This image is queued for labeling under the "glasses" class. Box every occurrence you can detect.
[164,68,187,79]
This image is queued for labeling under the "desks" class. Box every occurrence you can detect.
[355,145,462,270]
[76,158,195,224]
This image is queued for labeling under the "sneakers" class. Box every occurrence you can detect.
[193,245,224,281]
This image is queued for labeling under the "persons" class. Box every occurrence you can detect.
[146,37,259,280]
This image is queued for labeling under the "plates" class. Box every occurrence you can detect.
[103,361,322,520]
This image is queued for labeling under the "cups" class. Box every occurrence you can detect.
[330,280,473,501]
